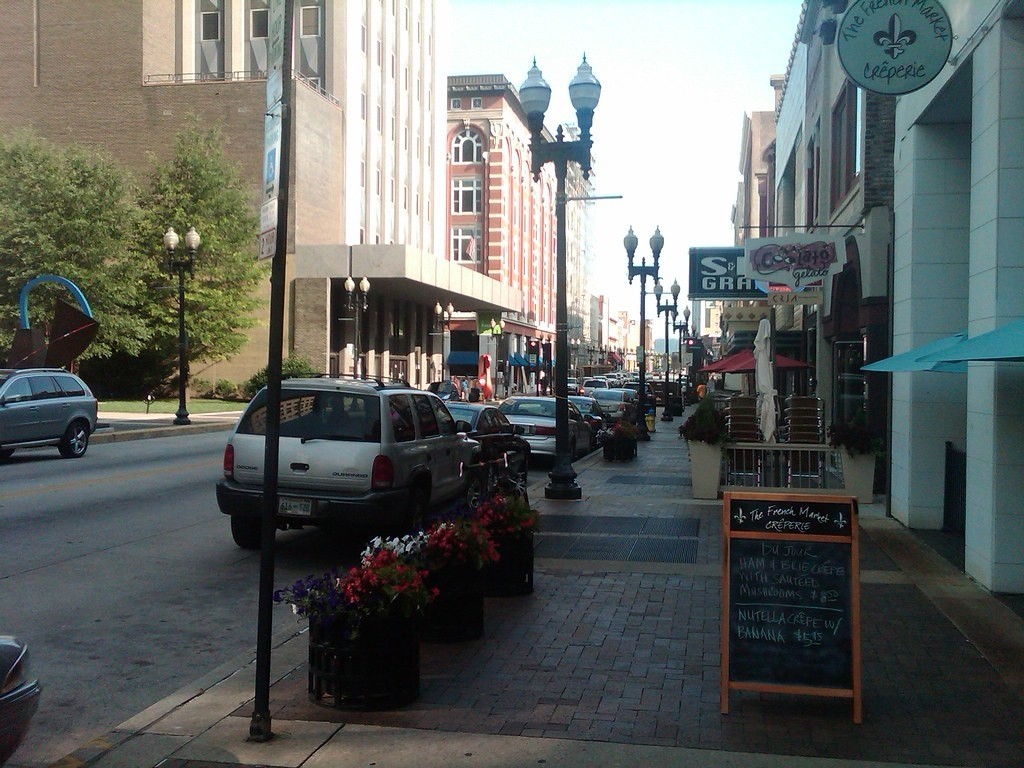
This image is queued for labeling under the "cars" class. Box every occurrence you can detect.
[443,371,655,483]
[0,634,43,764]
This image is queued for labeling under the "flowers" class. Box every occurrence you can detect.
[467,493,539,535]
[277,551,436,621]
[598,422,639,439]
[827,417,888,456]
[677,396,733,443]
[360,518,500,580]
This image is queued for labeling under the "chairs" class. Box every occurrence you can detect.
[319,409,349,432]
[724,392,826,487]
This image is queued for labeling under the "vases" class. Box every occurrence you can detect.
[304,605,427,708]
[419,569,487,641]
[839,443,877,503]
[603,438,637,460]
[479,533,534,598]
[685,440,721,500]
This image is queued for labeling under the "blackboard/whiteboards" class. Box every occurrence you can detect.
[720,492,860,698]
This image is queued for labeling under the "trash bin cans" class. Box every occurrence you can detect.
[471,388,480,402]
[669,396,682,416]
[645,394,656,416]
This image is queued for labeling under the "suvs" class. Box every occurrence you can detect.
[0,365,100,461]
[215,373,491,549]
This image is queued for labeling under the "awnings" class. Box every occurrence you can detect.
[447,351,478,366]
[524,355,536,366]
[515,354,529,366]
[508,354,519,366]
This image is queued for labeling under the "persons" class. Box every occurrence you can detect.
[454,375,469,402]
[696,383,707,399]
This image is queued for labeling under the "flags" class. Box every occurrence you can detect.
[467,236,475,262]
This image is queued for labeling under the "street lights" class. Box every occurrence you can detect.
[654,278,691,421]
[517,49,602,500]
[344,276,370,380]
[624,225,664,439]
[434,302,455,383]
[489,318,506,400]
[163,226,202,425]
[570,338,581,369]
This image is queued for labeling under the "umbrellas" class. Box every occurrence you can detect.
[860,331,968,373]
[752,319,777,452]
[695,348,815,396]
[914,318,1024,363]
